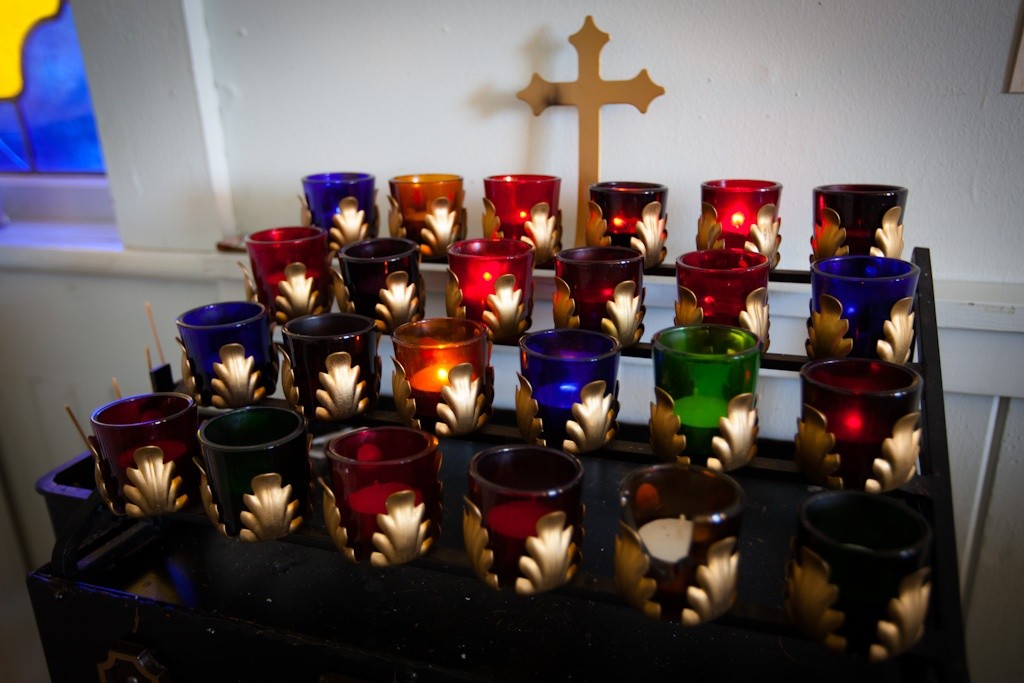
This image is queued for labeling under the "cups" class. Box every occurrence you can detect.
[90,171,935,666]
